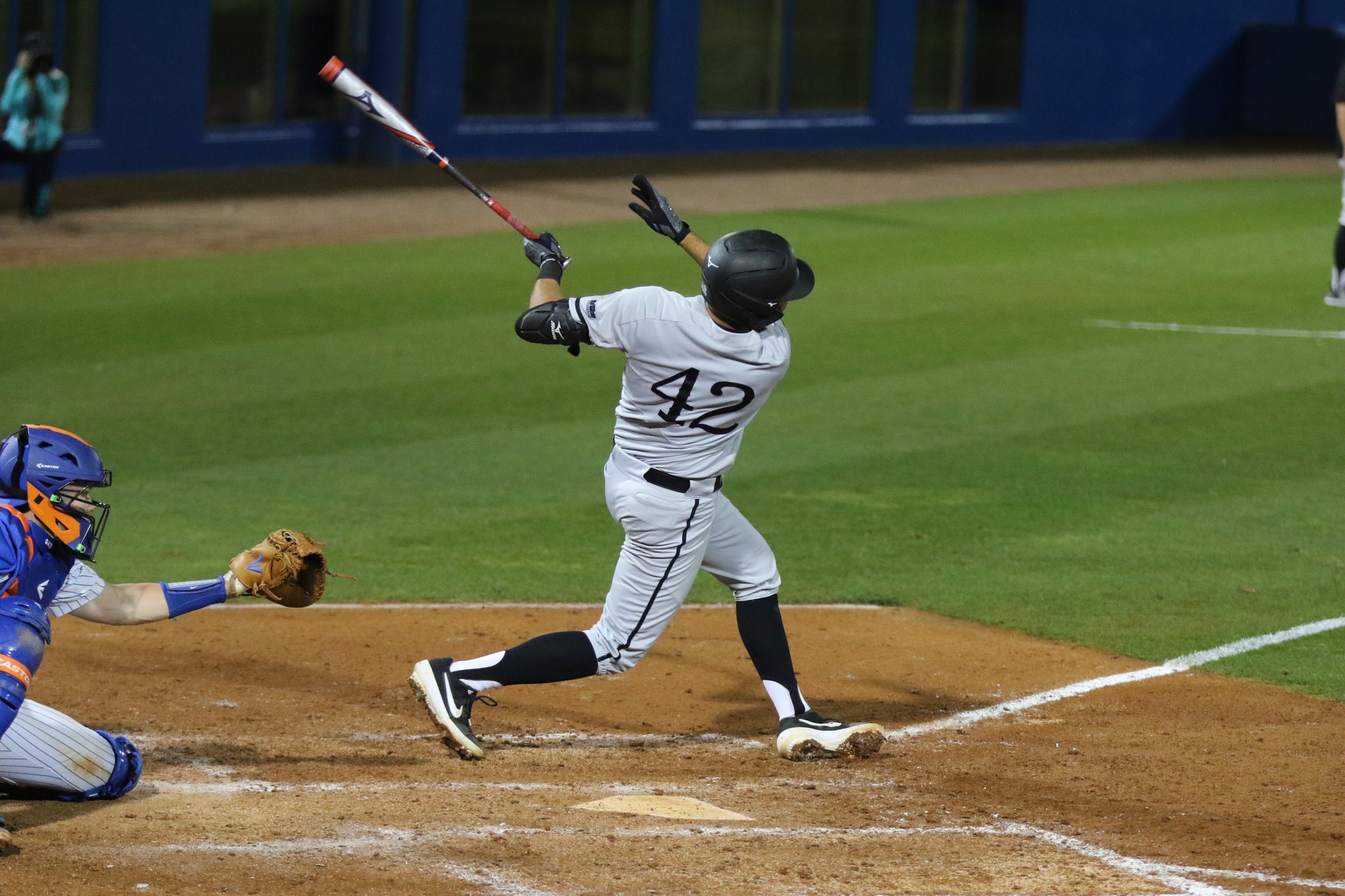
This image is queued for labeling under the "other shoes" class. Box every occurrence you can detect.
[1324,292,1345,306]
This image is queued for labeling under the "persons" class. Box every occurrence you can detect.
[1323,58,1345,308]
[408,172,887,763]
[0,422,330,853]
[0,45,69,220]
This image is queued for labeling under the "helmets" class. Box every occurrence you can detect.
[701,229,816,333]
[0,424,113,563]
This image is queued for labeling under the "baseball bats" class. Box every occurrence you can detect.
[319,53,572,272]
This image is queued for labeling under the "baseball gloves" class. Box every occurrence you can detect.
[225,527,358,607]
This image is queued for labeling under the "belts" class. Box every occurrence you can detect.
[643,468,723,494]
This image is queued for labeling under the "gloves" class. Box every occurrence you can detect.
[523,230,564,286]
[628,174,690,245]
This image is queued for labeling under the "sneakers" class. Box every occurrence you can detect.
[776,709,884,761]
[406,657,498,761]
